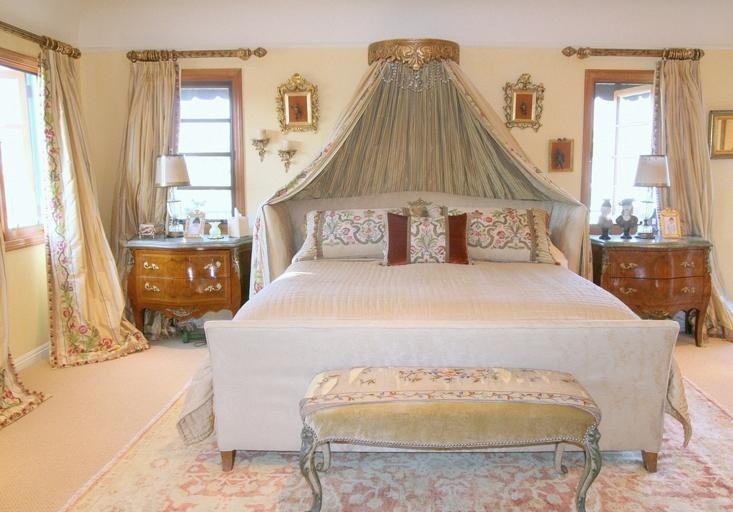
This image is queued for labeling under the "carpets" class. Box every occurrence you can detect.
[59,377,733,512]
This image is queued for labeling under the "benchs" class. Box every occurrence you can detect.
[299,368,602,512]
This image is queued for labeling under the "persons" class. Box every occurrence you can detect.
[190,218,201,234]
[169,220,178,232]
[666,218,676,234]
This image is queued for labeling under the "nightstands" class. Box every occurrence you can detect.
[590,235,714,347]
[123,235,253,334]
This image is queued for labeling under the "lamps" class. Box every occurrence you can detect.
[634,155,670,239]
[154,153,191,237]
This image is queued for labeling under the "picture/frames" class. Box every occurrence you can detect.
[708,110,733,160]
[660,207,682,239]
[275,72,320,135]
[503,73,546,132]
[548,137,576,173]
[184,209,205,241]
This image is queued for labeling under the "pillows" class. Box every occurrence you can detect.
[382,213,473,265]
[423,206,569,267]
[292,208,426,262]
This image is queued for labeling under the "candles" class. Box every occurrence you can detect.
[258,128,266,139]
[282,141,292,151]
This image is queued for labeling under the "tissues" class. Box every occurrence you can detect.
[228,206,248,238]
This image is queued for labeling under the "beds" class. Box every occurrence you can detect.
[178,192,692,473]
